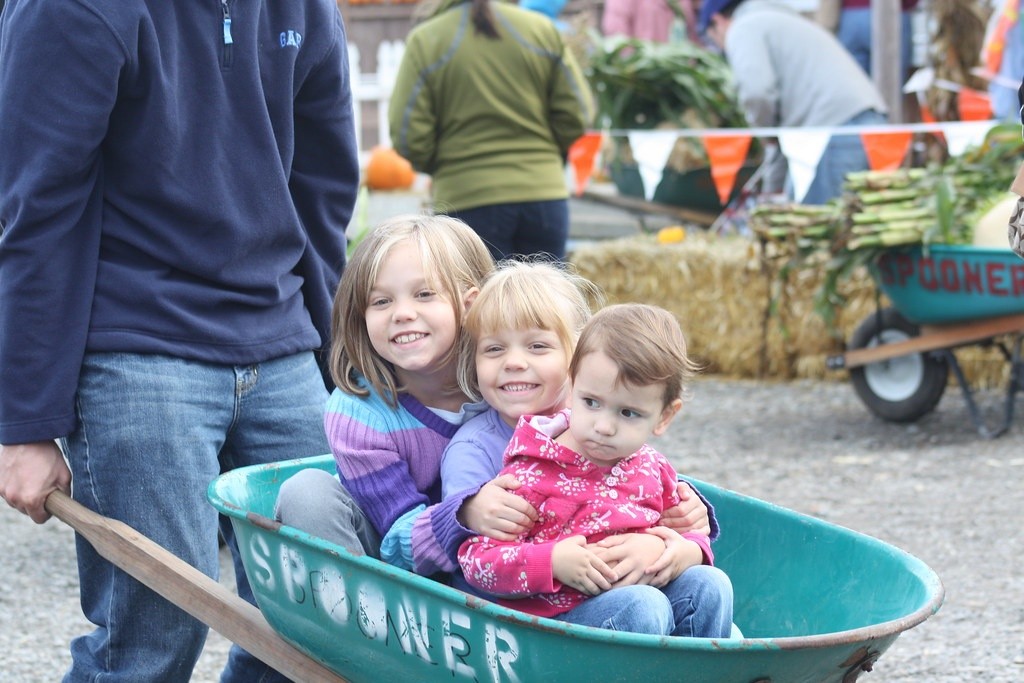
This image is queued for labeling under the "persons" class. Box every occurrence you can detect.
[692,0,895,229]
[454,300,735,639]
[0,0,388,683]
[275,213,721,611]
[439,262,711,609]
[381,0,603,275]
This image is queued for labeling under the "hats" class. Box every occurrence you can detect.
[694,0,733,34]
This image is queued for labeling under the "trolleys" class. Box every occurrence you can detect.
[41,433,941,683]
[843,239,1023,430]
[605,141,770,234]
